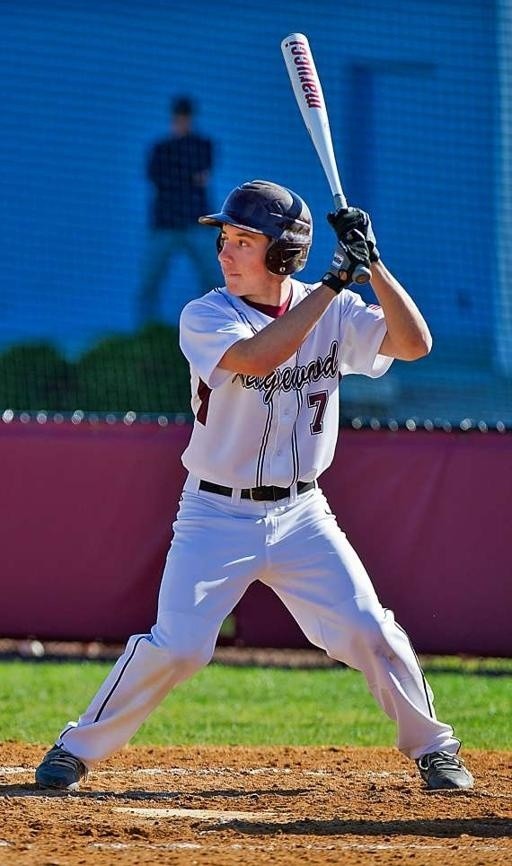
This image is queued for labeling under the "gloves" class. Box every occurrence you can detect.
[320,228,370,295]
[326,206,380,263]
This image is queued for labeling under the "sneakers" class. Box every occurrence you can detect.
[34,743,90,793]
[415,752,474,790]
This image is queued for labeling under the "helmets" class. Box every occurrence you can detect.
[198,178,315,276]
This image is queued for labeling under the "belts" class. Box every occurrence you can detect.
[199,479,316,501]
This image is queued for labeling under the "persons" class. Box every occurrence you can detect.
[136,87,226,328]
[37,176,475,794]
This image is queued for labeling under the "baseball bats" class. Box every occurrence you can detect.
[279,31,372,284]
